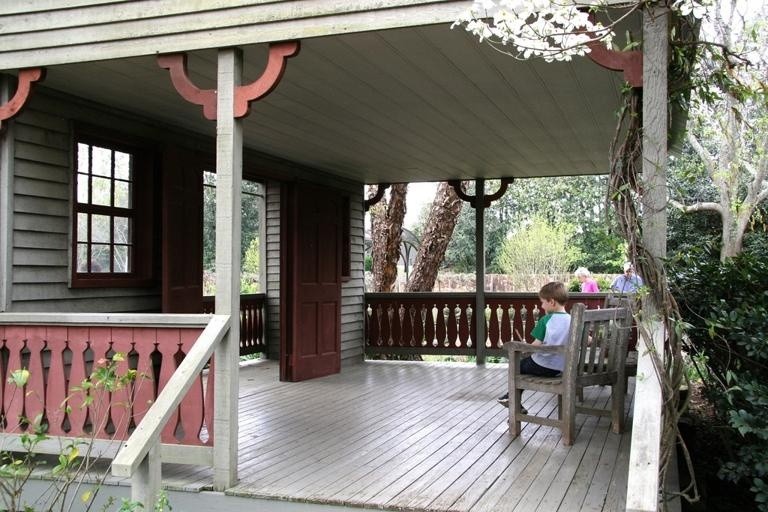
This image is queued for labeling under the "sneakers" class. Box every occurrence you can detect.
[506,406,528,426]
[496,392,511,403]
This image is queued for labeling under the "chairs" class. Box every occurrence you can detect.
[503,294,638,446]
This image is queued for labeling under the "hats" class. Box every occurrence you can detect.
[624,261,635,271]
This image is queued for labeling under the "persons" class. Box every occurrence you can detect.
[610,260,644,352]
[574,266,601,347]
[495,281,594,425]
